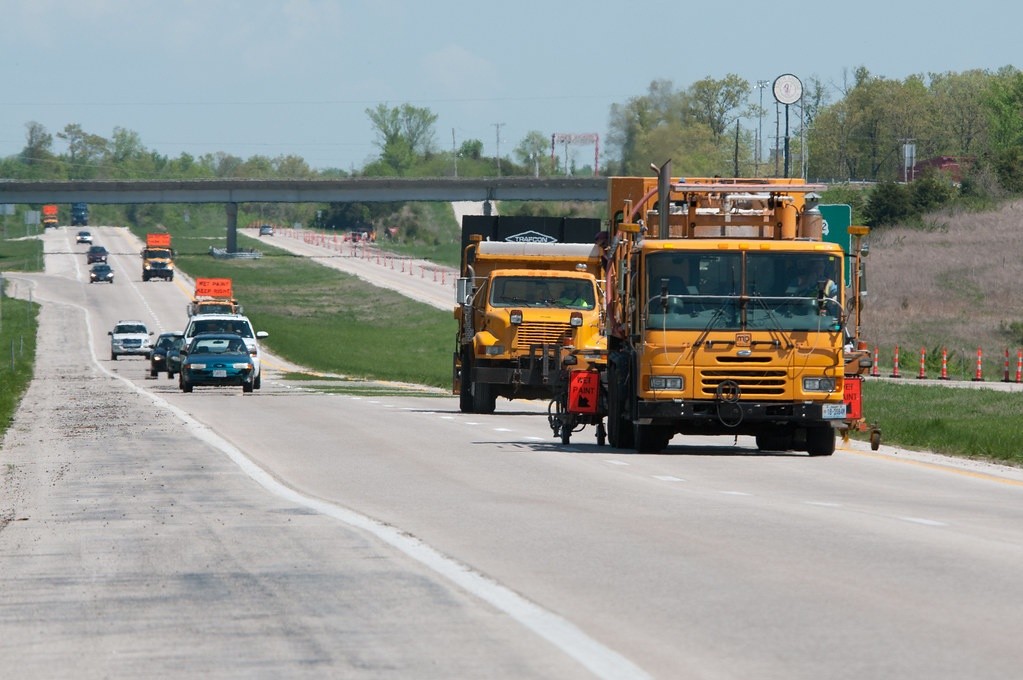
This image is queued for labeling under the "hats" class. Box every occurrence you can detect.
[564,282,578,291]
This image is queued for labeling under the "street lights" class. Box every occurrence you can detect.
[752,78,770,164]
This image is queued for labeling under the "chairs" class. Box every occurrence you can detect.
[197,346,209,352]
[651,277,690,312]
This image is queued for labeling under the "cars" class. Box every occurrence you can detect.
[180,335,257,393]
[89,264,114,283]
[148,333,183,376]
[164,336,185,379]
[260,225,273,237]
[86,247,108,265]
[77,231,92,245]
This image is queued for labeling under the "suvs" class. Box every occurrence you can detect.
[173,312,269,390]
[107,319,154,360]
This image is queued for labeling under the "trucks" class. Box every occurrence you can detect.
[42,213,60,228]
[71,202,90,227]
[140,246,175,282]
[547,158,882,457]
[451,215,610,413]
[186,294,243,318]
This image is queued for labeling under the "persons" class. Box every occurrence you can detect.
[557,283,589,307]
[226,340,243,354]
[796,256,837,318]
[356,224,401,243]
